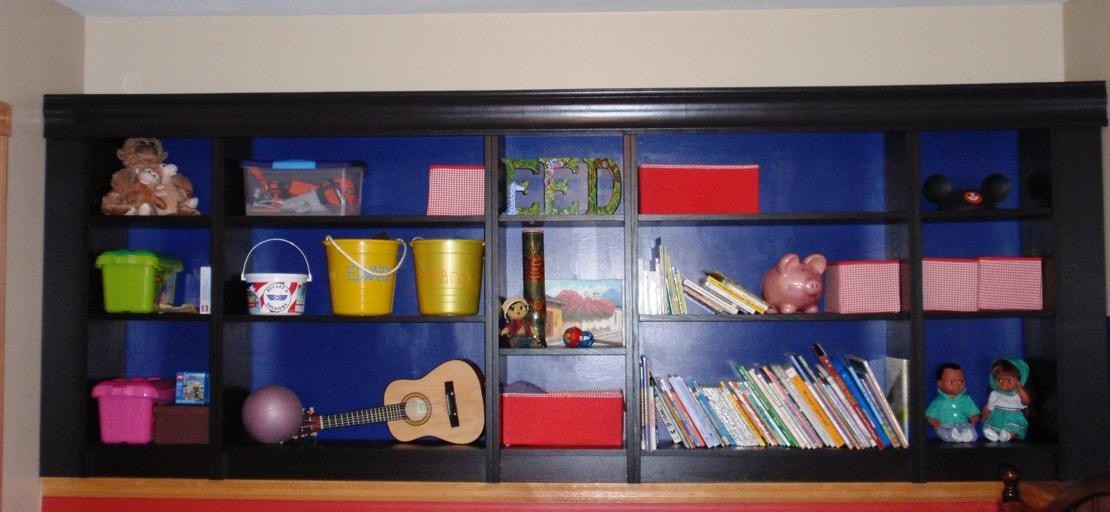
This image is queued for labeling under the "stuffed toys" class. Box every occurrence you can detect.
[101,137,200,215]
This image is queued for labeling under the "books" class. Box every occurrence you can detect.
[637,237,769,315]
[639,342,911,451]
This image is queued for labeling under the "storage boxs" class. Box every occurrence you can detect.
[922,259,978,312]
[640,164,759,214]
[241,160,365,215]
[502,390,625,448]
[97,249,184,312]
[978,257,1043,310]
[95,377,175,443]
[823,259,901,313]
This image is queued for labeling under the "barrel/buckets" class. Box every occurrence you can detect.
[410,236,483,315]
[241,237,311,316]
[322,234,406,316]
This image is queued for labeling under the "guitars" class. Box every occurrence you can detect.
[292,358,485,444]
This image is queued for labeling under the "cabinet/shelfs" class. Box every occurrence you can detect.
[38,89,1109,483]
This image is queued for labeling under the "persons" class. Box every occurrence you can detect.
[923,362,981,442]
[500,297,544,348]
[981,358,1032,442]
